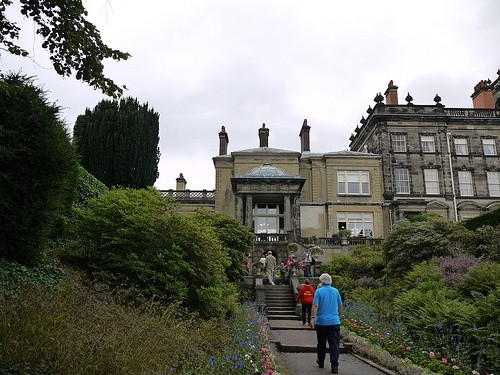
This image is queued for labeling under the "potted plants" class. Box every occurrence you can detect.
[287,243,298,256]
[337,228,353,245]
[252,262,264,275]
[307,245,325,261]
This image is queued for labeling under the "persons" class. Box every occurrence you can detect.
[310,273,342,374]
[296,279,315,325]
[266,251,312,285]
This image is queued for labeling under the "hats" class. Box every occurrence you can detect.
[319,273,332,284]
[268,251,272,253]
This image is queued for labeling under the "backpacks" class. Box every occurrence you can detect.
[311,257,315,265]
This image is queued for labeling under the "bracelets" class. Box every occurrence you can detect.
[310,317,315,319]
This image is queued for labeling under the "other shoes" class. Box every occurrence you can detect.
[317,360,324,368]
[331,367,338,373]
